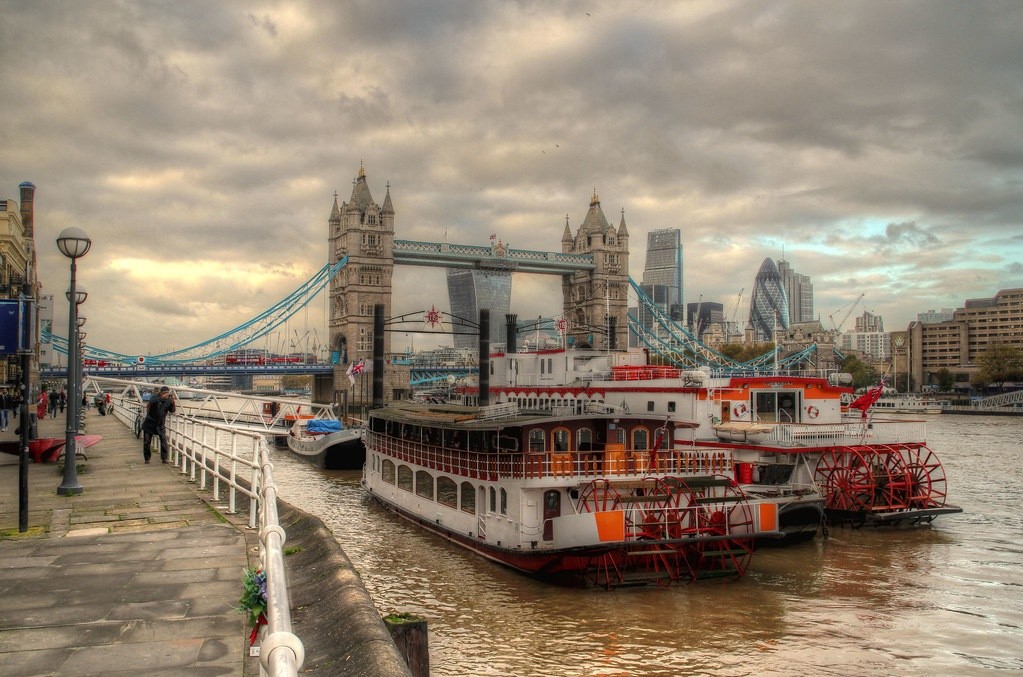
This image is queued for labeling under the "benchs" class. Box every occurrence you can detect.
[56,445,88,463]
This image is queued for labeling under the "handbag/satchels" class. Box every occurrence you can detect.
[36,398,43,406]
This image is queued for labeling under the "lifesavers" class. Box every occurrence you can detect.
[808,405,819,419]
[734,403,746,417]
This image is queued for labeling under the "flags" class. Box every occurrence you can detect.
[347,358,363,376]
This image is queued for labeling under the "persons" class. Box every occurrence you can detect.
[0,387,12,432]
[58,389,66,413]
[49,389,59,418]
[141,386,177,465]
[11,391,20,419]
[81,396,92,411]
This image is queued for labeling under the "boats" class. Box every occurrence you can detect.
[704,361,963,544]
[286,417,368,469]
[361,309,782,591]
[870,397,945,414]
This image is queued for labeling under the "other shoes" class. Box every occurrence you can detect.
[5,426,8,431]
[145,458,151,463]
[163,459,168,463]
[1,427,5,432]
[50,414,52,418]
[54,415,56,418]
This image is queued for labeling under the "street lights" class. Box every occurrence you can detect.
[56,226,93,496]
[65,284,88,429]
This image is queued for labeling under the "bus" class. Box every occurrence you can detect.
[226,352,273,366]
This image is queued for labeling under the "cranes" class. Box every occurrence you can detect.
[695,291,704,334]
[723,288,746,321]
[829,292,867,332]
[270,327,329,364]
